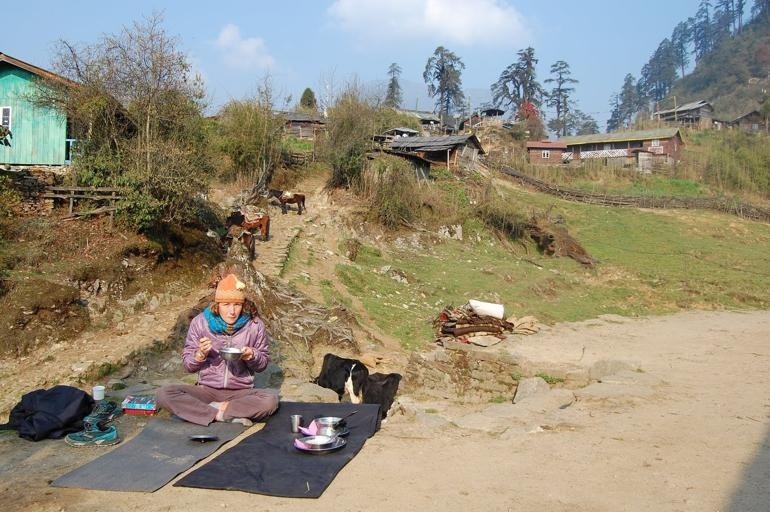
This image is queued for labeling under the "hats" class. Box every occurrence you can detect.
[213,274,247,304]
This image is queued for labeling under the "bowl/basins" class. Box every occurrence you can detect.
[219,347,242,362]
[316,417,345,430]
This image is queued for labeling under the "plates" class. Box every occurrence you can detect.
[294,427,349,455]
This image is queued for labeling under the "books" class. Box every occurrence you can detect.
[123,408,159,417]
[120,394,160,411]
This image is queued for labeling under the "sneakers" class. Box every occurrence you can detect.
[63,426,120,447]
[82,400,120,424]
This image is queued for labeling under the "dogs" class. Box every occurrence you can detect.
[309,353,369,405]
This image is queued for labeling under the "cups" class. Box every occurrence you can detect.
[93,386,105,400]
[291,414,304,433]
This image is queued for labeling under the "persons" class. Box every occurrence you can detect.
[153,272,280,429]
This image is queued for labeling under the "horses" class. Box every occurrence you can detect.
[225,211,270,242]
[218,229,255,262]
[267,189,306,215]
